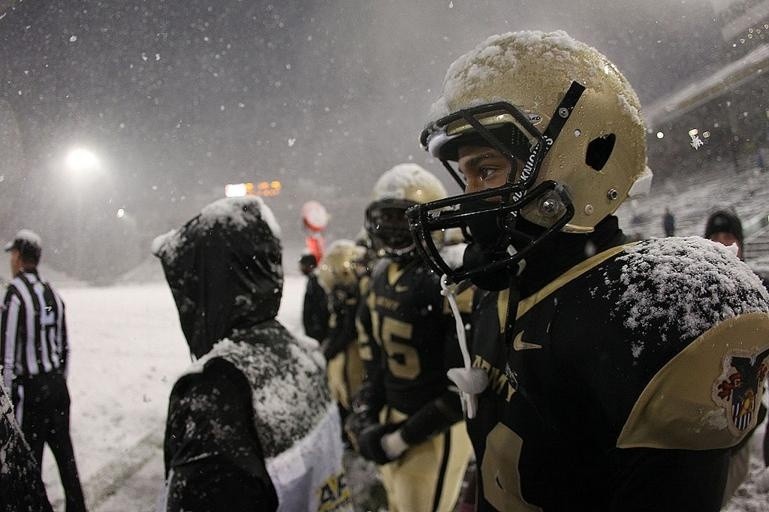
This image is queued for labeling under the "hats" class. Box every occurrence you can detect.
[706,209,743,244]
[2,229,44,268]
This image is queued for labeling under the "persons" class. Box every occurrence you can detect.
[297,166,480,512]
[0,378,51,511]
[704,210,745,262]
[663,209,676,237]
[148,191,354,512]
[0,225,89,511]
[403,26,769,510]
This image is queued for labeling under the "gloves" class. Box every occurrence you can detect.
[341,404,407,466]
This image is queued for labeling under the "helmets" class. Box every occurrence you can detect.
[417,29,649,243]
[364,162,452,255]
